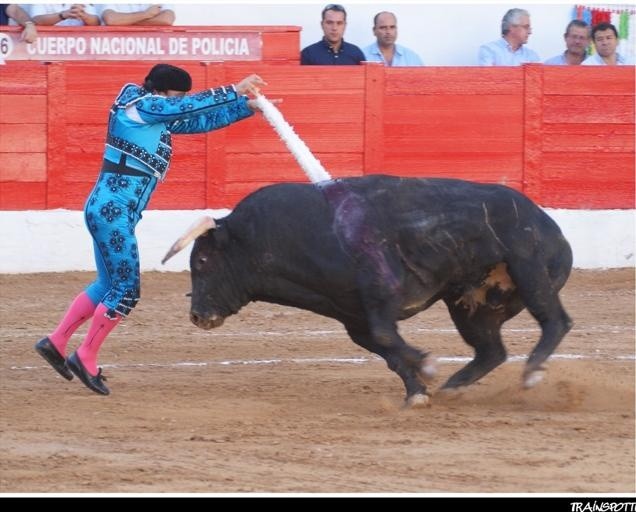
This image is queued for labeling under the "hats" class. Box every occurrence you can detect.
[143,62,193,92]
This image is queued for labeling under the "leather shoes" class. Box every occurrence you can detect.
[34,336,73,380]
[64,349,109,396]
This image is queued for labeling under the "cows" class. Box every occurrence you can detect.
[159,173,575,408]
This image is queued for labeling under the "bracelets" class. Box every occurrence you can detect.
[26,20,35,26]
[60,10,66,20]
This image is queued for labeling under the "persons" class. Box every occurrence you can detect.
[29,4,103,26]
[300,4,365,64]
[544,20,592,65]
[579,21,634,65]
[36,63,282,396]
[0,4,38,43]
[100,3,177,26]
[360,11,425,66]
[478,7,542,66]
